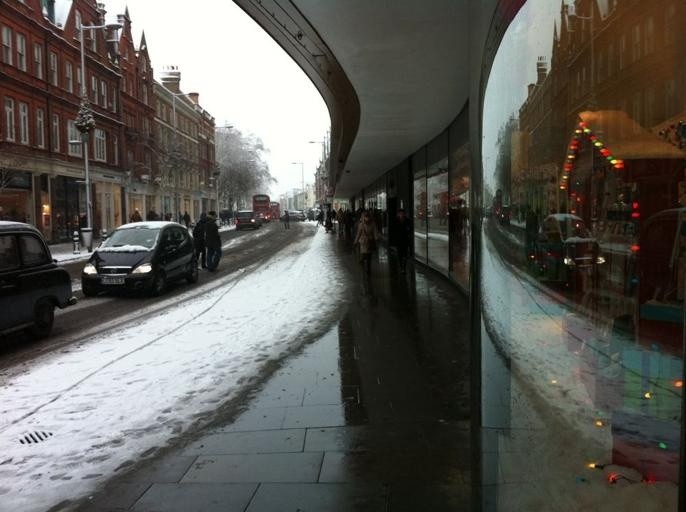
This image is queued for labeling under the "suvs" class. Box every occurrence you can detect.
[236,210,263,229]
[499,204,511,228]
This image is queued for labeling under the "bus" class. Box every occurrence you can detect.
[496,189,503,216]
[252,195,272,221]
[270,202,281,220]
[432,190,449,217]
[492,196,497,214]
[417,191,426,220]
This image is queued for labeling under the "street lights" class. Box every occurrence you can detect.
[200,116,233,222]
[70,19,123,252]
[292,161,305,209]
[568,12,599,113]
[172,90,199,223]
[208,172,222,227]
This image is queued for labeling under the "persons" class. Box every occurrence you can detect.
[280,207,389,242]
[353,211,382,275]
[128,206,234,231]
[389,209,415,275]
[487,187,570,265]
[205,211,222,273]
[192,211,208,268]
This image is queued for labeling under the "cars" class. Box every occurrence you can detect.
[597,221,639,260]
[82,220,199,298]
[0,221,78,339]
[622,204,684,327]
[526,214,605,277]
[282,210,307,222]
